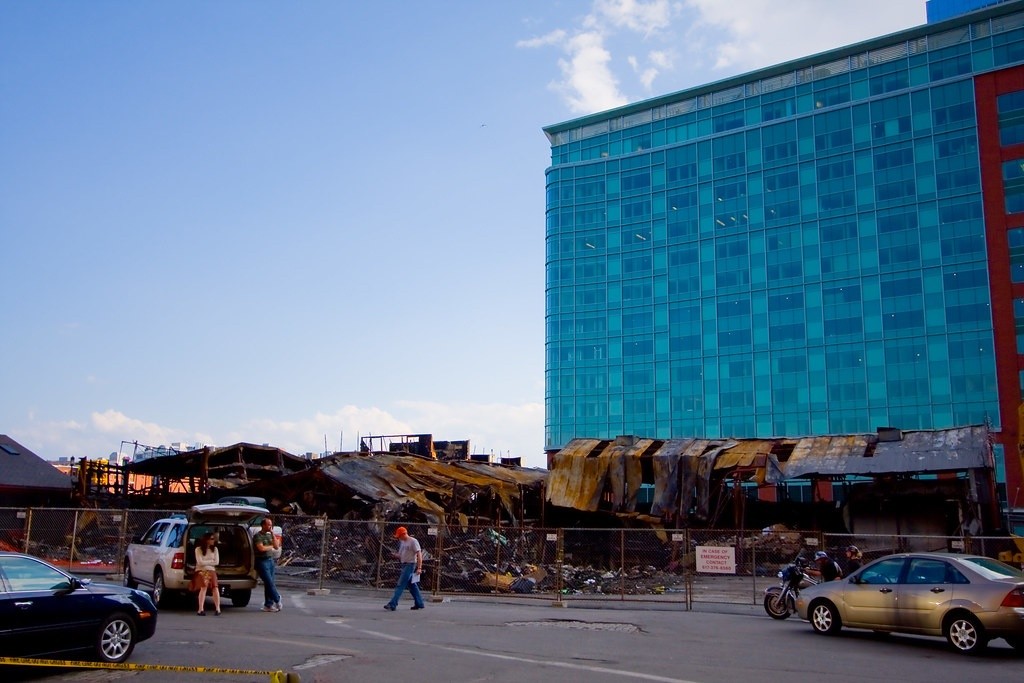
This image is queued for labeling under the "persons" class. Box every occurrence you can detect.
[833,545,864,580]
[804,551,840,582]
[194,532,222,616]
[376,526,424,611]
[252,518,283,612]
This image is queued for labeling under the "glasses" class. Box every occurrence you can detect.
[210,538,215,540]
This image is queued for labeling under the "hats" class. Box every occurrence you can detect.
[393,526,407,537]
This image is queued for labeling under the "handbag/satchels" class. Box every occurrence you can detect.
[271,547,281,558]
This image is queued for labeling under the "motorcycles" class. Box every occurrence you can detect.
[763,549,825,620]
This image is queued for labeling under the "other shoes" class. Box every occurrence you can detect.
[411,604,424,610]
[197,610,206,616]
[384,604,395,611]
[215,611,221,615]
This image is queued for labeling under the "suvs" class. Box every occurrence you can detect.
[121,503,270,607]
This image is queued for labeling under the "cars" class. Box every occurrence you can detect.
[0,551,159,673]
[795,552,1024,657]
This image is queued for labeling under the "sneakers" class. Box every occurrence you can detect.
[275,595,282,610]
[262,604,279,611]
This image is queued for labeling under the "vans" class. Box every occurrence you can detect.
[218,497,266,508]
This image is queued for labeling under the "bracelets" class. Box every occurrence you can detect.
[418,567,421,569]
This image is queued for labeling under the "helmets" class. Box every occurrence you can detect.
[846,545,859,553]
[814,551,828,560]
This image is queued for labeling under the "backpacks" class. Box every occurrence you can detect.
[830,561,843,577]
